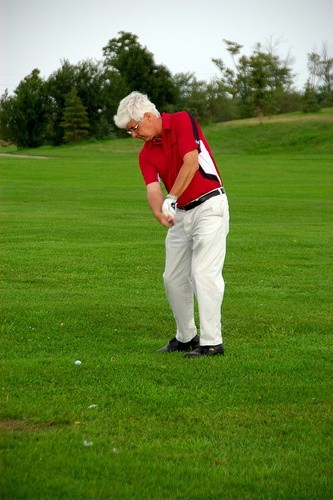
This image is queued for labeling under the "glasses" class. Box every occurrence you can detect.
[126,119,141,135]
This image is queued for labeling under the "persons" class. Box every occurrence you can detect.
[111,91,230,358]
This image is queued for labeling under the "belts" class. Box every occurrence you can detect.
[179,188,226,211]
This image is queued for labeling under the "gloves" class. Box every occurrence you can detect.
[161,194,177,220]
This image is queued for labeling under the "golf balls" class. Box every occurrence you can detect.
[74,360,82,365]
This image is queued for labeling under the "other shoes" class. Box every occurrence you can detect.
[185,343,224,357]
[156,335,199,353]
